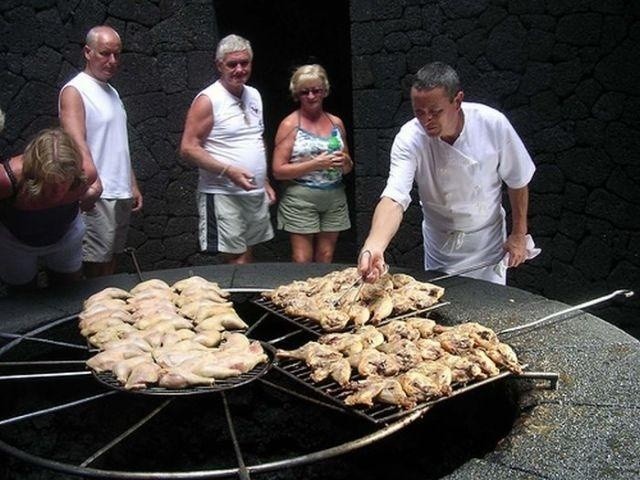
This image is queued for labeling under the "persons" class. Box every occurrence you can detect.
[180,34,279,264]
[0,127,97,296]
[272,64,354,264]
[57,25,145,277]
[356,61,539,286]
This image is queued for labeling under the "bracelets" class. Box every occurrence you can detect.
[219,164,229,178]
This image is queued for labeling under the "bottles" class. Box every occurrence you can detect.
[325,130,343,181]
